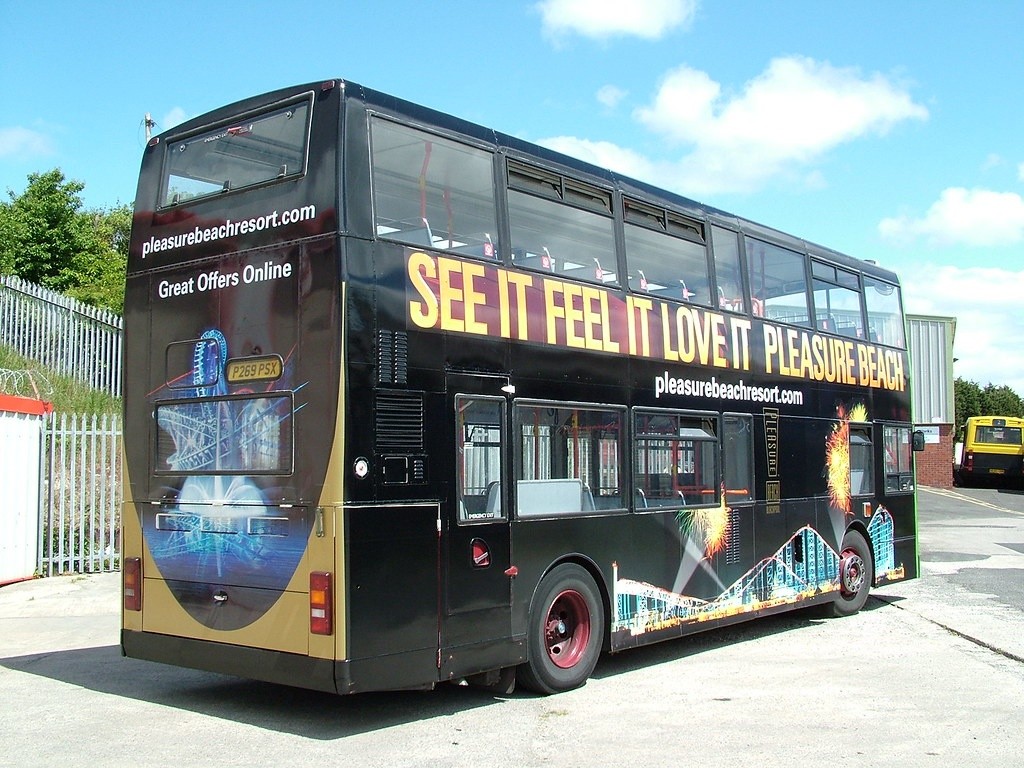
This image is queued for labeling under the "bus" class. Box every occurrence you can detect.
[119,78,924,697]
[958,415,1024,487]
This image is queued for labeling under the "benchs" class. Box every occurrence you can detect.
[603,269,649,293]
[486,478,584,517]
[552,255,605,284]
[858,327,879,342]
[486,481,596,511]
[687,286,727,309]
[590,486,648,510]
[647,279,690,301]
[433,231,498,260]
[774,313,838,333]
[511,243,554,273]
[378,217,434,247]
[612,489,686,507]
[836,320,858,338]
